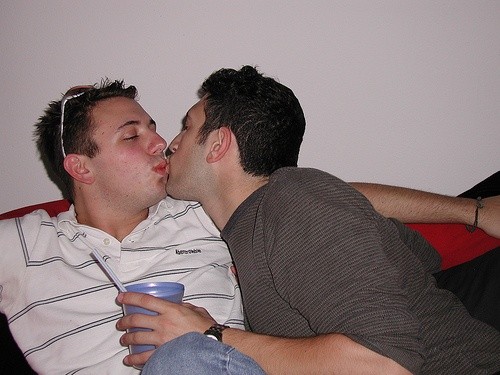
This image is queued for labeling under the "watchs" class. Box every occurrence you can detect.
[201,324,232,346]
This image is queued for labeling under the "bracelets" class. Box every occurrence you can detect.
[465,197,484,234]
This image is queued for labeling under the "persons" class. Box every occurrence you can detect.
[115,68,499,374]
[0,82,499,375]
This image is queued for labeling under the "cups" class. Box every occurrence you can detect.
[118,282,185,371]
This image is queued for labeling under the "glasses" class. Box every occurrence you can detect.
[60,84,98,159]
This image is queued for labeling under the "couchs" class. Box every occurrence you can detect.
[0,200,499,275]
